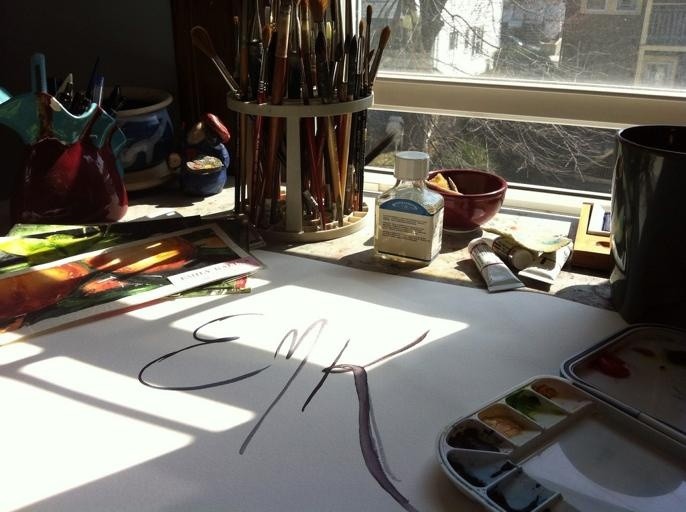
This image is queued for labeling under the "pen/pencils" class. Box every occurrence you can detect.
[29,52,126,120]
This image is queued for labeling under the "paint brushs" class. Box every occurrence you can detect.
[192,0,391,232]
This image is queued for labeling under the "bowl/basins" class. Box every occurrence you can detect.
[424,168,508,234]
